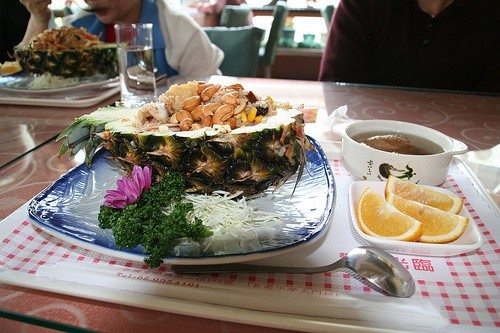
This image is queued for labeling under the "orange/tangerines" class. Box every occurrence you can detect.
[388,193,470,243]
[385,175,464,217]
[358,187,423,242]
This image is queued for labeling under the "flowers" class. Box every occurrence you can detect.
[102,165,151,208]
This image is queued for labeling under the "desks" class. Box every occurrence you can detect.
[0,74,500,333]
[249,1,322,17]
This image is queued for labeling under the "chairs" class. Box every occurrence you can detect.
[204,1,289,77]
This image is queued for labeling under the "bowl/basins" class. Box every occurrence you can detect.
[333,120,469,186]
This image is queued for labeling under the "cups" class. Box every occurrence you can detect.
[115,23,158,108]
[304,34,315,42]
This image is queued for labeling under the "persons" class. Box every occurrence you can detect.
[318,0,500,94]
[188,0,253,27]
[19,0,225,76]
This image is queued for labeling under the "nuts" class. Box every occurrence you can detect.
[171,80,245,131]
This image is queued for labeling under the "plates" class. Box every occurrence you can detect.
[0,76,120,97]
[349,180,483,257]
[27,134,337,264]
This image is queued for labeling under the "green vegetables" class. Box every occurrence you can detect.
[97,173,214,271]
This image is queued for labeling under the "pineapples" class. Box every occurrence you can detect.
[13,42,124,81]
[57,106,311,198]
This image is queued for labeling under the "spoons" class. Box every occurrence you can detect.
[71,0,107,12]
[172,246,416,298]
